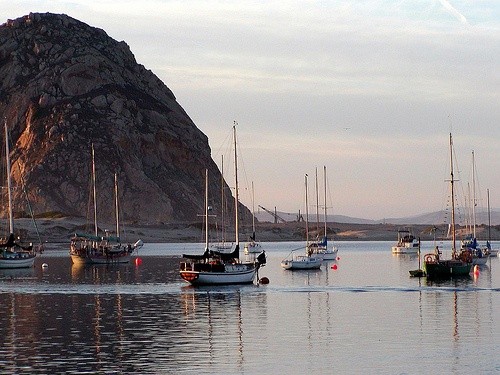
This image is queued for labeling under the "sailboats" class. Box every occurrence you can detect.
[281,174,324,271]
[0,116,47,268]
[69,142,145,264]
[179,120,269,285]
[424,115,475,282]
[457,149,492,265]
[479,187,499,257]
[391,225,421,254]
[307,165,339,261]
